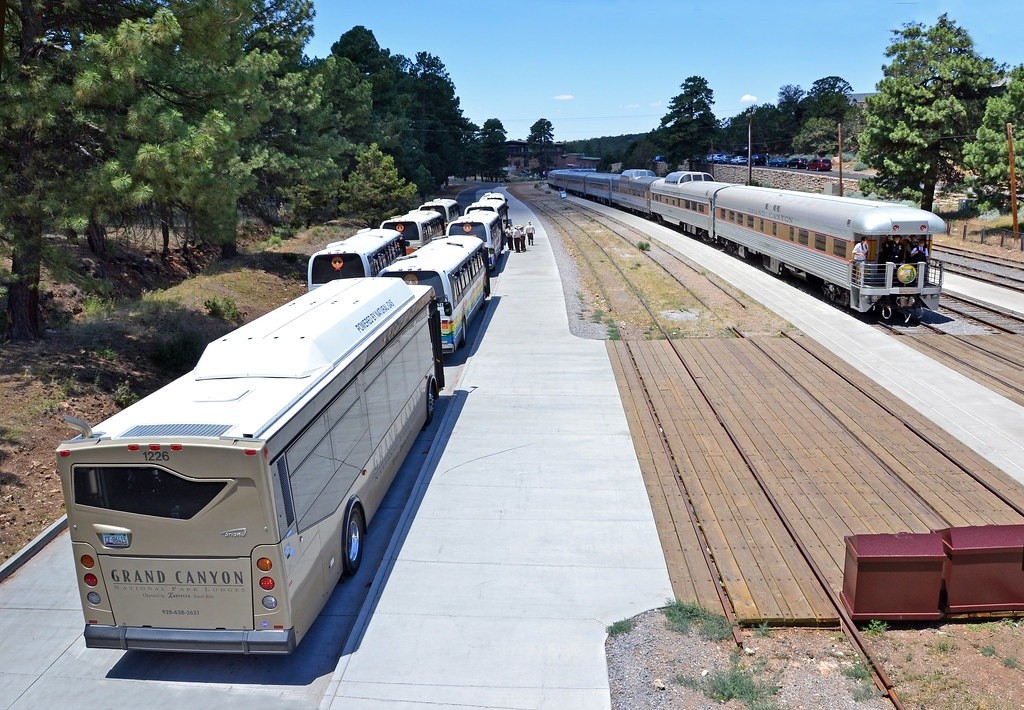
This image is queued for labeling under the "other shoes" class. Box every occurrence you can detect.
[509,248,526,253]
[528,242,530,245]
[532,243,533,245]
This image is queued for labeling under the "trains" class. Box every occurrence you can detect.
[548,168,948,322]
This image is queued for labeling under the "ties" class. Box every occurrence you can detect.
[921,247,922,251]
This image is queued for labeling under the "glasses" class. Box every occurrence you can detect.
[919,242,924,244]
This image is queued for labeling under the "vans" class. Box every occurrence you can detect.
[806,158,832,171]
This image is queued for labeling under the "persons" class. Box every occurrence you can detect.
[882,236,929,286]
[505,224,514,250]
[852,236,869,283]
[514,226,520,253]
[519,226,526,252]
[525,222,536,245]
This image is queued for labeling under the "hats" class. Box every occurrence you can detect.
[514,225,523,229]
[908,235,917,238]
[529,221,531,222]
[892,235,902,238]
[508,224,510,226]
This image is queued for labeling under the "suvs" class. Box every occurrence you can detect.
[707,154,723,163]
[784,158,809,169]
[746,155,766,166]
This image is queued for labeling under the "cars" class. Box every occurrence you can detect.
[730,156,747,165]
[718,155,731,164]
[768,158,787,167]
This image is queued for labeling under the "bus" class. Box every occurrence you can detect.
[446,209,506,269]
[377,235,495,355]
[464,199,508,232]
[480,192,508,206]
[307,228,410,292]
[380,209,444,255]
[419,197,460,231]
[51,277,446,656]
